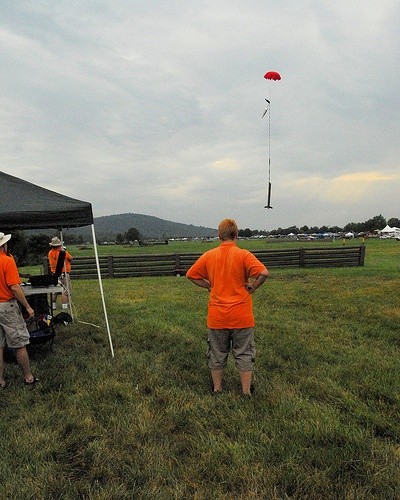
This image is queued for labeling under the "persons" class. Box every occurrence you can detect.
[186,219,269,396]
[48,237,73,310]
[0,232,40,388]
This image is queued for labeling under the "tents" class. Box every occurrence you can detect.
[251,225,400,239]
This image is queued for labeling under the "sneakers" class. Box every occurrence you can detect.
[24,376,40,384]
[0,381,9,390]
[61,304,68,310]
[53,303,57,310]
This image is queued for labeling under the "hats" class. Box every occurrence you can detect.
[0,233,11,246]
[49,236,63,246]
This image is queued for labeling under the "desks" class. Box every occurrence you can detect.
[21,284,64,322]
